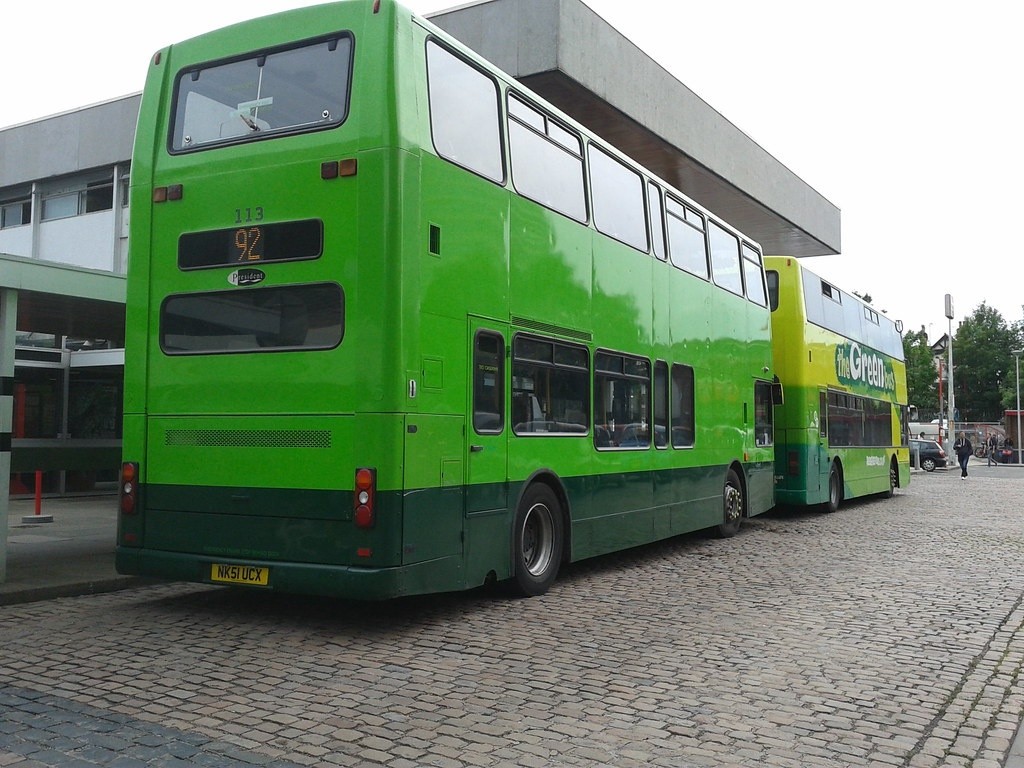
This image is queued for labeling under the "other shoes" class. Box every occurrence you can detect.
[961,476,968,480]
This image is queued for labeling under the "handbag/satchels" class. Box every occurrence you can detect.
[1002,449,1012,456]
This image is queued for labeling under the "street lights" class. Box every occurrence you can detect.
[935,356,946,447]
[1011,350,1022,464]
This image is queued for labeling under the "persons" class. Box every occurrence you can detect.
[984,432,1014,468]
[953,433,973,479]
[917,432,926,440]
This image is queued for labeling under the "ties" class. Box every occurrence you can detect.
[961,438,963,446]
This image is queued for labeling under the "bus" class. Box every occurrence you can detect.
[113,5,781,601]
[759,255,912,515]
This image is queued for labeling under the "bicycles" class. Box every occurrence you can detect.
[974,442,1005,458]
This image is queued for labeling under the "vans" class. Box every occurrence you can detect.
[908,423,939,442]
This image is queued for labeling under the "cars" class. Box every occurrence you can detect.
[908,439,947,472]
